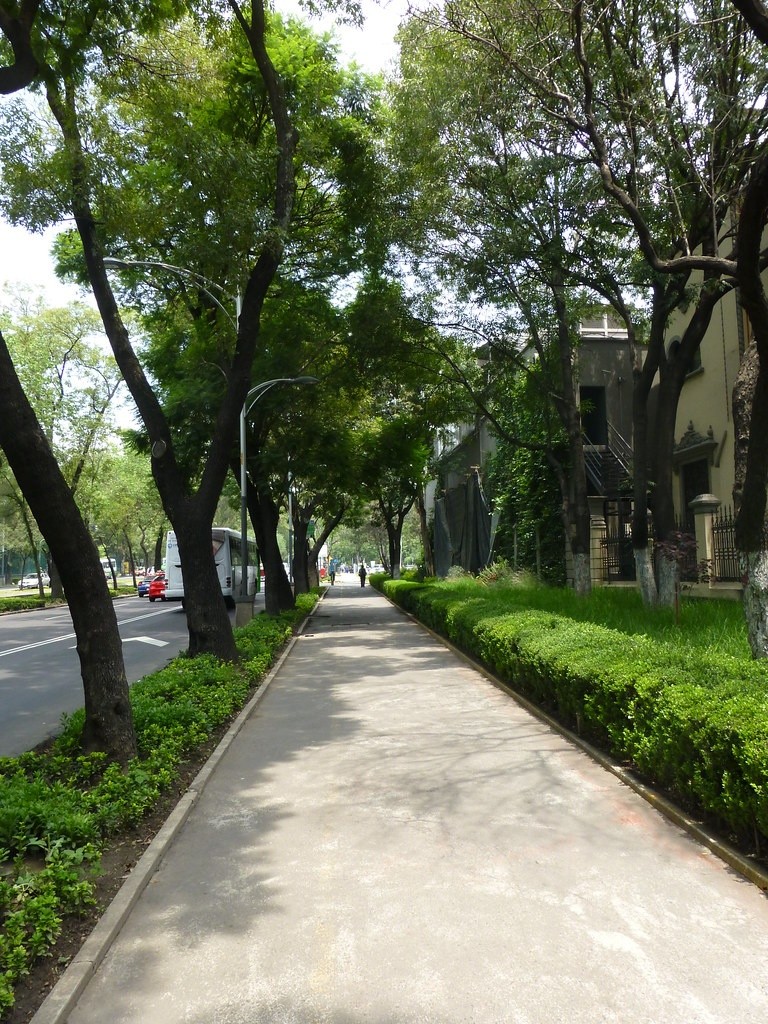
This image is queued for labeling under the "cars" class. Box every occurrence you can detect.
[138,575,156,597]
[149,572,166,602]
[18,573,50,589]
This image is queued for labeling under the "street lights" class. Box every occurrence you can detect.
[287,464,326,585]
[105,258,318,627]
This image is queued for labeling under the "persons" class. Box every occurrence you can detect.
[359,565,366,587]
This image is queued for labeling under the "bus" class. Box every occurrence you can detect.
[99,559,117,579]
[164,527,261,609]
